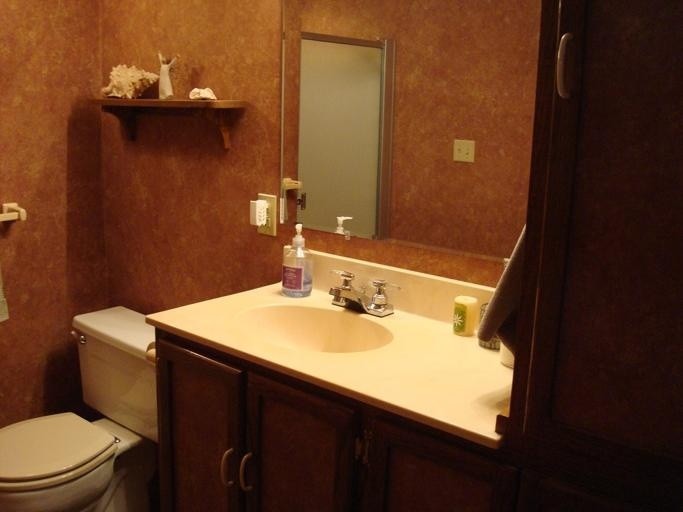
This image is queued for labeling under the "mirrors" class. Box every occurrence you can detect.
[281,1,540,266]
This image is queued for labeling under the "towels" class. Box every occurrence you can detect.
[477,219,526,355]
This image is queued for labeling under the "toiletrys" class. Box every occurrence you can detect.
[282,222,313,296]
[335,214,353,234]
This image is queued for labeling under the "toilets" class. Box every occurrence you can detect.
[0,303,158,509]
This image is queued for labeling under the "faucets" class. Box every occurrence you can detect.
[325,264,395,318]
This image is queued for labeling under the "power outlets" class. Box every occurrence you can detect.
[250,192,278,237]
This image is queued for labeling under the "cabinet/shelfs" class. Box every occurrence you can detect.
[356,413,593,511]
[158,338,353,510]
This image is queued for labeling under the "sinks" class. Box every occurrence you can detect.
[227,299,397,357]
